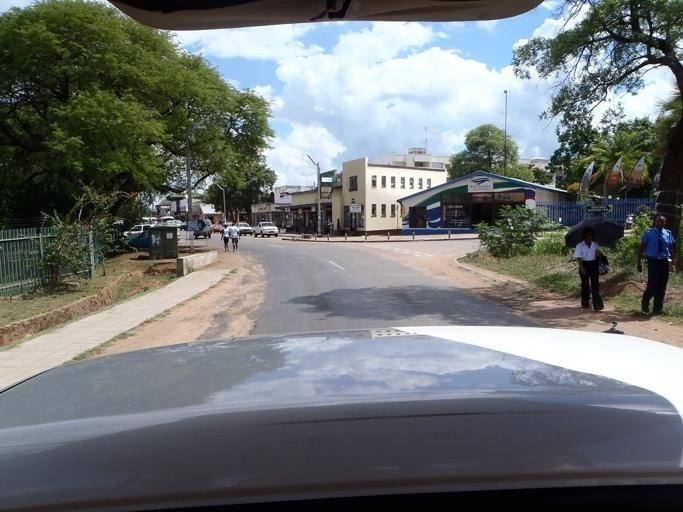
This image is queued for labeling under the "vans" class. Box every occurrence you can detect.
[193,219,213,239]
[115,213,188,244]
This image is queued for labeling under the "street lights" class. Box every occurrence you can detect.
[502,89,511,176]
[216,184,227,224]
[306,154,323,237]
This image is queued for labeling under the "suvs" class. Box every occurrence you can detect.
[236,222,253,236]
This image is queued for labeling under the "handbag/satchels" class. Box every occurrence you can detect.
[599,260,608,275]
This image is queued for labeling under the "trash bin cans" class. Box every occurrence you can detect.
[148,227,178,259]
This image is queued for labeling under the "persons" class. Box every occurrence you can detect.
[637,213,676,315]
[221,223,230,253]
[575,232,607,312]
[230,223,240,252]
[337,218,342,237]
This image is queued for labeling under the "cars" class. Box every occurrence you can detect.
[212,224,225,232]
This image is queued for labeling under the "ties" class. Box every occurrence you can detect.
[658,231,663,254]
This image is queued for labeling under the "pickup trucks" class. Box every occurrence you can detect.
[254,222,279,238]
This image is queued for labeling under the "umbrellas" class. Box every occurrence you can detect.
[563,216,625,250]
